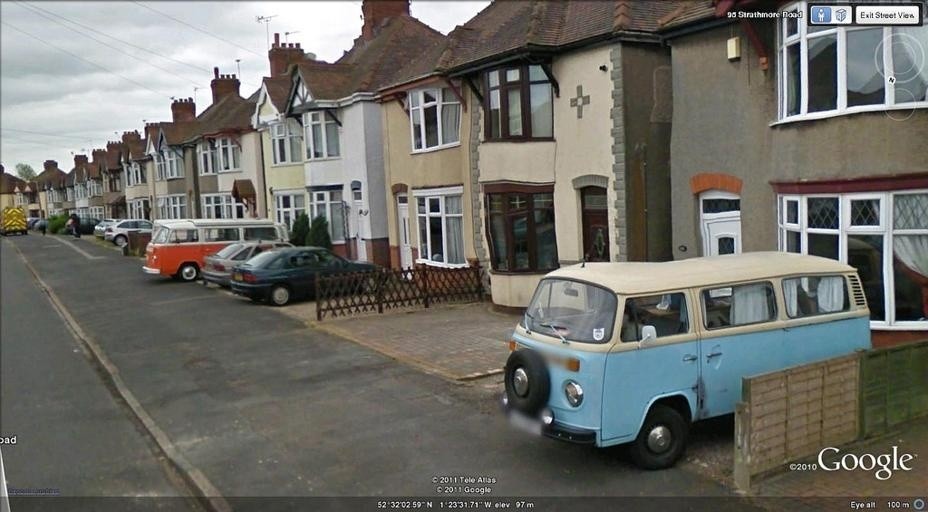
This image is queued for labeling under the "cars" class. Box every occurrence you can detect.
[230,246,388,307]
[200,240,295,287]
[95,219,122,236]
[104,219,153,247]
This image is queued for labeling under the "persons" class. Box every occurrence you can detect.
[41,216,46,234]
[71,214,80,237]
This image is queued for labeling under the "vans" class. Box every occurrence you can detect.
[141,218,289,282]
[501,250,871,472]
